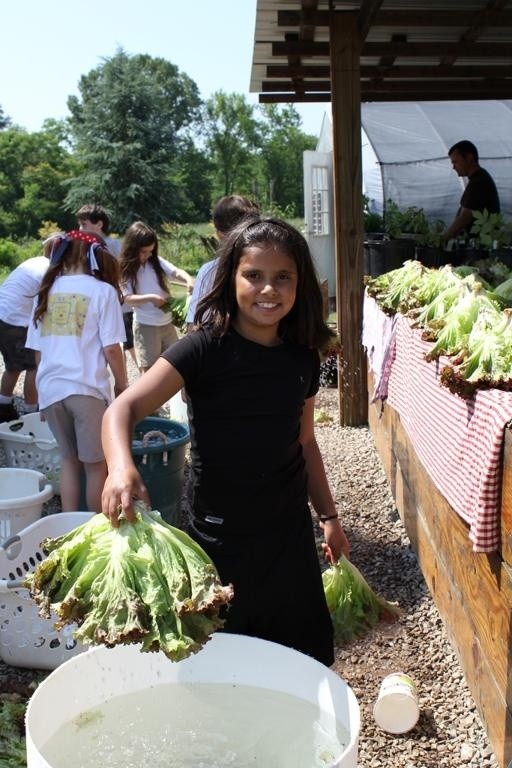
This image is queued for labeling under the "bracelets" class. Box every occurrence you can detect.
[318,512,338,525]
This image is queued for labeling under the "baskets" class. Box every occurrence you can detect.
[0,410,103,670]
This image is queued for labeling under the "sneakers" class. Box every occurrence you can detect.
[0,402,19,422]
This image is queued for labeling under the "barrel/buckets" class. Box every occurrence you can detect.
[26,631,361,768]
[131,416,190,529]
[364,231,443,280]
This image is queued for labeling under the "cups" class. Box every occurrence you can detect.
[373,673,422,735]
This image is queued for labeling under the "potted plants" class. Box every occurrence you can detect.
[363,195,512,279]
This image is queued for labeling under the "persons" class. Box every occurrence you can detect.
[436,138,502,265]
[184,192,261,333]
[117,217,197,420]
[0,232,66,421]
[73,200,141,376]
[98,216,353,667]
[24,229,130,514]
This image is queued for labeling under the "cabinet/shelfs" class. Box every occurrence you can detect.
[361,282,510,657]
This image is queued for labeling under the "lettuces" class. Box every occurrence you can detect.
[23,498,234,663]
[363,258,512,397]
[160,291,194,332]
[322,552,407,652]
